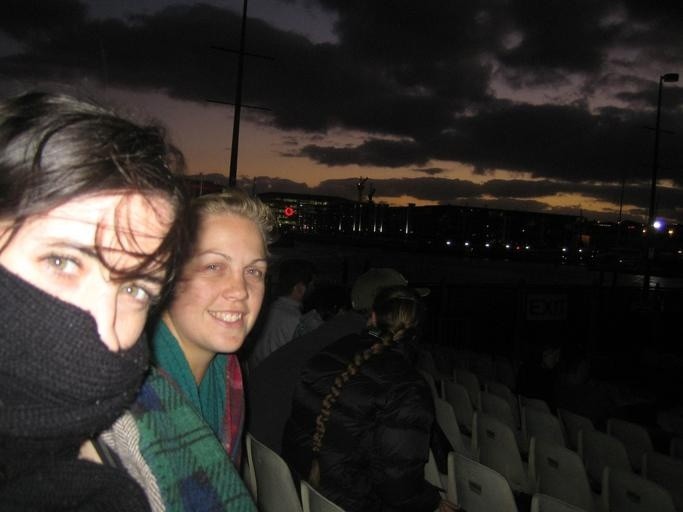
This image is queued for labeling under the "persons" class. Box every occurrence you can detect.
[0,74,200,510]
[283,280,463,512]
[74,185,280,510]
[243,261,436,457]
[238,253,324,372]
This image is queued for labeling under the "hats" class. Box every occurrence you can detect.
[350,266,431,312]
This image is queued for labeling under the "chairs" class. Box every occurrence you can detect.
[419,365,680,511]
[244,431,302,510]
[298,479,345,511]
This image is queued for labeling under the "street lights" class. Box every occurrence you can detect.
[640,72,680,306]
